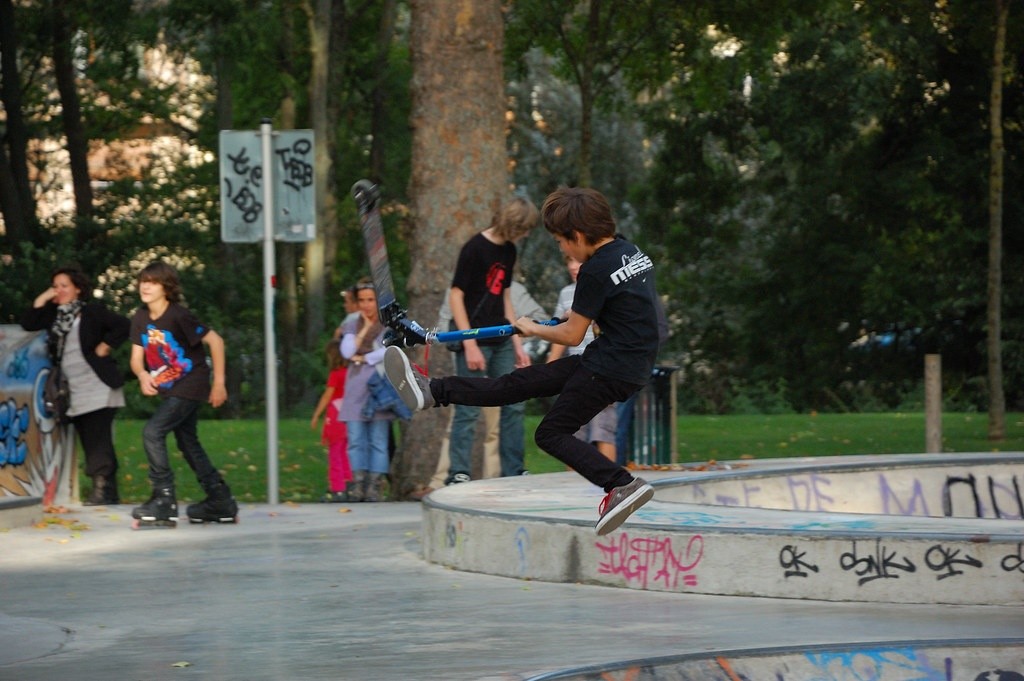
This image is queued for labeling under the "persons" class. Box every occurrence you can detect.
[383,187,659,537]
[405,197,669,502]
[309,274,400,503]
[128,262,237,519]
[21,269,131,506]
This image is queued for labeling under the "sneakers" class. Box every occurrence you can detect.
[595,476,654,536]
[384,345,436,413]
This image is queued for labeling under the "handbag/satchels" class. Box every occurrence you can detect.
[447,312,480,351]
[44,365,70,419]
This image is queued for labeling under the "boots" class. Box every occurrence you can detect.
[81,468,119,505]
[364,473,382,501]
[347,471,368,501]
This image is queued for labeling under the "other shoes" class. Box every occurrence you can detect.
[410,487,435,500]
[444,472,471,486]
[321,491,347,502]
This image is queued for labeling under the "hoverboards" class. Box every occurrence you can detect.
[349,178,573,350]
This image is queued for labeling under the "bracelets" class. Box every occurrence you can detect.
[356,334,366,340]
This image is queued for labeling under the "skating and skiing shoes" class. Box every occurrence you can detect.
[132,484,179,529]
[186,485,239,525]
